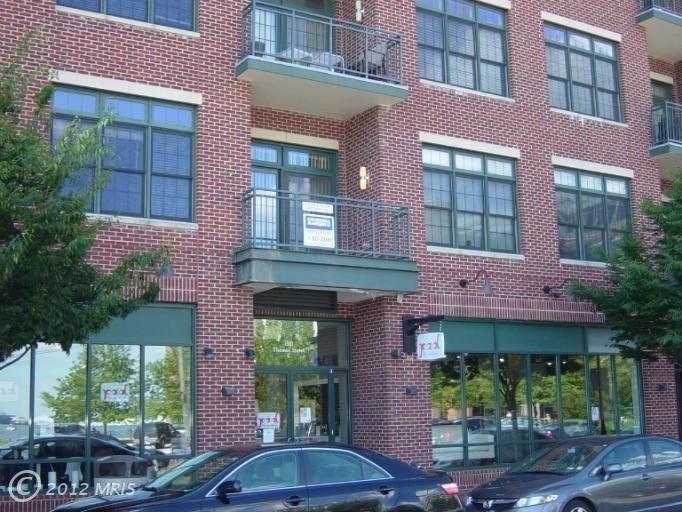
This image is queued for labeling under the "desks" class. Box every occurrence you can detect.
[276,46,344,73]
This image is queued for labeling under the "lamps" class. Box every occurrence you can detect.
[355,1,365,23]
[543,278,579,303]
[459,268,492,295]
[358,166,369,191]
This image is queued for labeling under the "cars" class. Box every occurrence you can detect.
[48,440,468,512]
[432,412,596,465]
[465,435,682,512]
[0,423,185,492]
[256,420,341,445]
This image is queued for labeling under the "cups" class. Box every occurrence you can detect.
[292,420,337,436]
[255,417,286,434]
[71,470,80,488]
[48,471,56,490]
[57,474,69,488]
[0,483,43,493]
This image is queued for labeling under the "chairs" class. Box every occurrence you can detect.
[346,41,389,82]
[248,462,297,489]
[318,463,361,483]
